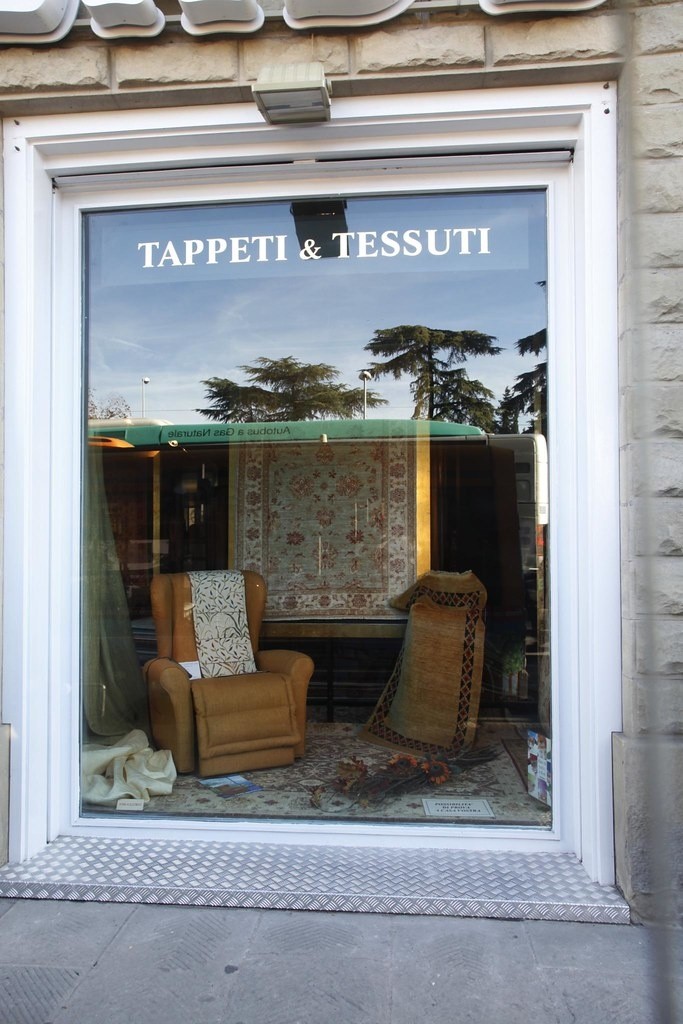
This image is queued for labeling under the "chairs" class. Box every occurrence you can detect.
[144,569,313,771]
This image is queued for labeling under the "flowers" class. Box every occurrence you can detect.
[309,752,452,813]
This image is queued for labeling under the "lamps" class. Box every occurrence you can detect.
[252,62,332,130]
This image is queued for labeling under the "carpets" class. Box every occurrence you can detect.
[357,569,487,760]
[91,722,551,824]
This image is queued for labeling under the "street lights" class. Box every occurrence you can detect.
[140,377,151,418]
[359,371,373,420]
